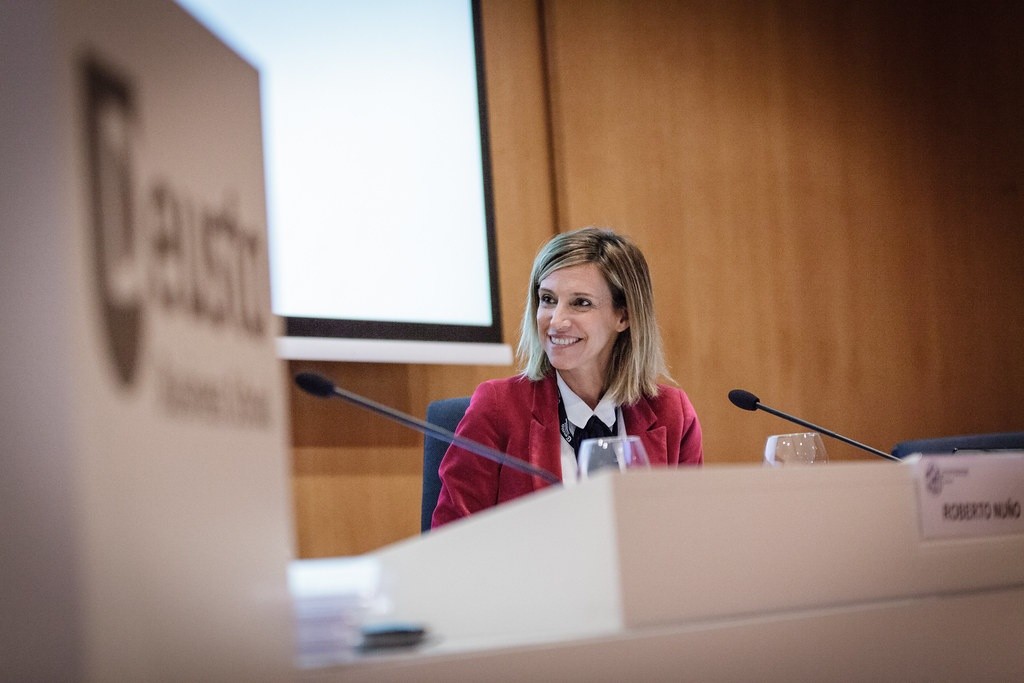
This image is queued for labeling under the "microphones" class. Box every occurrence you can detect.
[295,372,562,484]
[728,390,902,462]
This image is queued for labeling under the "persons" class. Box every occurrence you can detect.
[430,228,703,531]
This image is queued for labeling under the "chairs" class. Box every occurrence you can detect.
[419,396,469,531]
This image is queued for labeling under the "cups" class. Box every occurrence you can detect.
[579,437,651,480]
[764,432,830,466]
